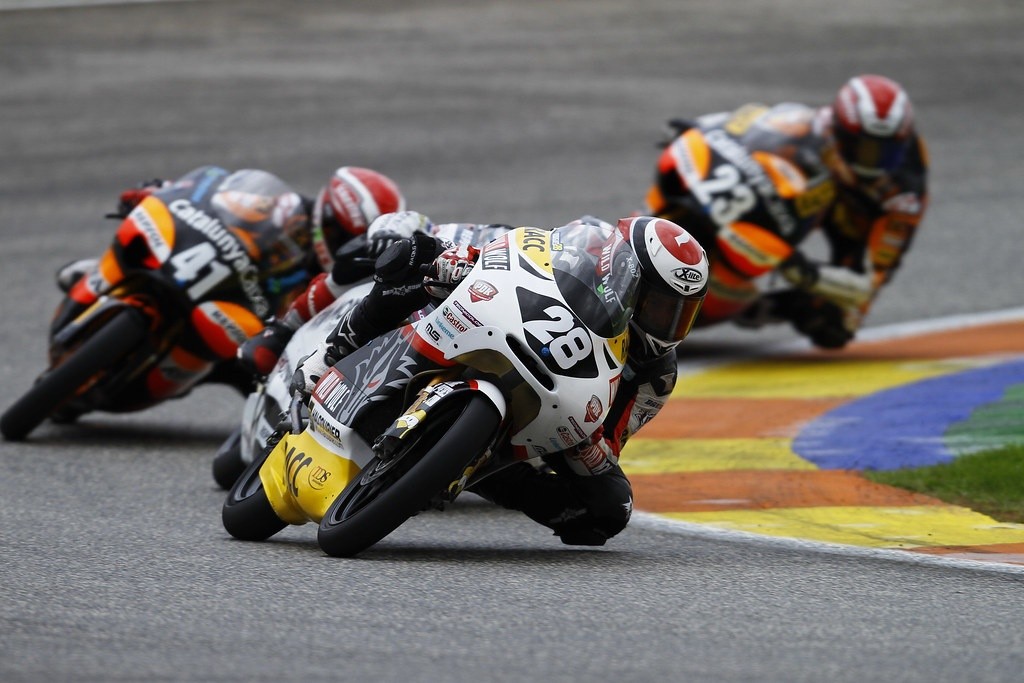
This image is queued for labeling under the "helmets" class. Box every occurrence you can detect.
[321,164,405,236]
[596,213,709,298]
[834,73,914,141]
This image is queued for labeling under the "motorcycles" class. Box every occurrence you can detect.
[218,221,644,557]
[2,162,307,445]
[651,101,842,331]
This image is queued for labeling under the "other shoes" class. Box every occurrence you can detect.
[235,317,293,380]
[291,342,337,396]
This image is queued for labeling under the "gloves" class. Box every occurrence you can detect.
[425,242,479,300]
[565,423,605,456]
[366,226,405,259]
[795,298,859,350]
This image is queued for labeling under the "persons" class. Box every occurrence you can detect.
[290,216,709,545]
[56,164,406,396]
[238,211,618,373]
[658,76,928,349]
